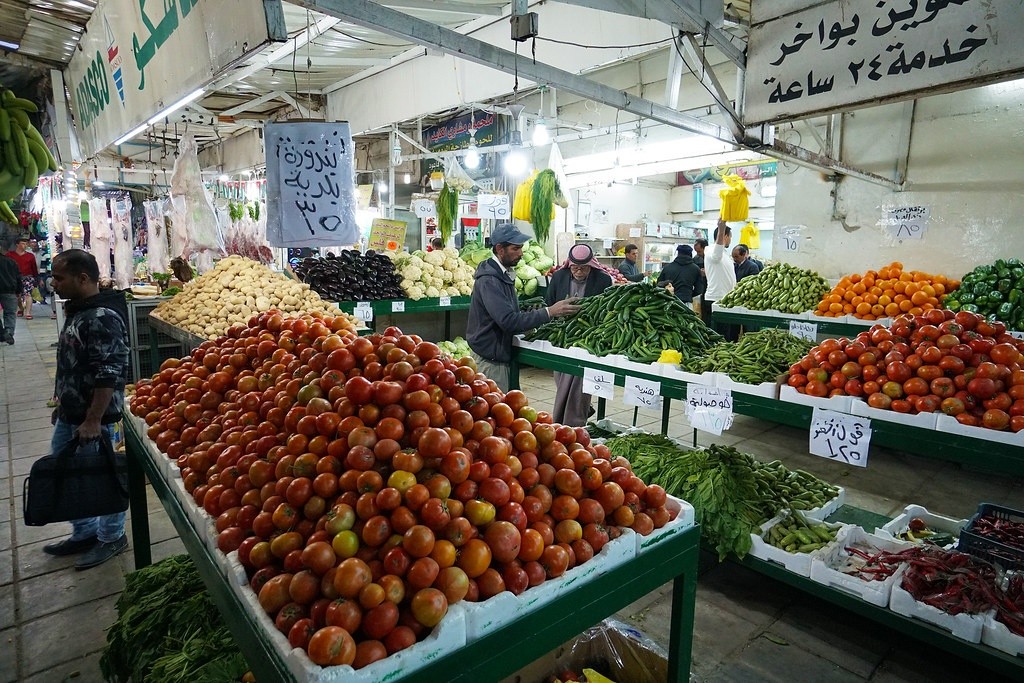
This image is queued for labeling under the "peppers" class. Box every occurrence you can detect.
[940,257,1024,332]
[680,325,819,385]
[841,513,1024,638]
[523,281,726,364]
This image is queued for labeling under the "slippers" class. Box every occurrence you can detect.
[17,308,23,317]
[26,315,33,320]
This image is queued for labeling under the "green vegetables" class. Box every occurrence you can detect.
[228,199,260,221]
[436,182,459,243]
[599,431,773,561]
[98,553,258,683]
[529,169,569,244]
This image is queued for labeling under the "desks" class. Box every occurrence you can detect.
[146,315,209,378]
[336,295,472,342]
[112,402,701,682]
[710,312,871,337]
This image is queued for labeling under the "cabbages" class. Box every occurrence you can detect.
[458,239,554,295]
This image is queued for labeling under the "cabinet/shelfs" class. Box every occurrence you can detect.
[618,224,708,279]
[509,346,1024,680]
[556,232,628,269]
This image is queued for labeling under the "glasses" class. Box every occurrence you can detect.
[571,266,589,272]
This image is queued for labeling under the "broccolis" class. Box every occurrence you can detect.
[380,248,477,301]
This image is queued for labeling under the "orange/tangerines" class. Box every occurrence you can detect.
[814,262,960,320]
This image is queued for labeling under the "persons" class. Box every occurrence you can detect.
[4,239,38,319]
[618,244,653,281]
[544,244,613,427]
[428,237,435,252]
[704,218,742,343]
[431,238,445,250]
[0,240,23,345]
[26,238,48,304]
[657,280,674,294]
[692,238,711,328]
[732,245,759,282]
[40,243,56,319]
[466,223,582,395]
[737,244,764,271]
[43,248,131,572]
[657,244,702,311]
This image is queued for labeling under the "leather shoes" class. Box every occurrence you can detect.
[75,532,128,570]
[44,533,98,555]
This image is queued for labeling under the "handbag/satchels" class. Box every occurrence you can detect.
[32,285,43,302]
[23,431,130,526]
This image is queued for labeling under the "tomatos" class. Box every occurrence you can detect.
[788,307,1024,433]
[131,310,678,670]
[242,671,256,683]
[909,517,925,530]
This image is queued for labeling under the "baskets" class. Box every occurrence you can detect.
[959,502,1024,571]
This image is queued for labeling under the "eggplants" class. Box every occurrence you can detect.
[295,248,409,302]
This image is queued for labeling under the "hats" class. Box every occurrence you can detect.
[490,223,532,246]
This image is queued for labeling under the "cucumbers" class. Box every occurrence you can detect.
[705,443,840,553]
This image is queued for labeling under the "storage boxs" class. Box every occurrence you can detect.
[124,299,1024,683]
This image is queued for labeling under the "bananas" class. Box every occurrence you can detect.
[0,88,59,226]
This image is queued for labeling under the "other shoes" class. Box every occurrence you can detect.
[40,300,47,305]
[4,331,14,345]
[51,313,56,319]
[589,406,595,417]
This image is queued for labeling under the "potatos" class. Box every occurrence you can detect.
[153,253,364,341]
[547,258,628,284]
[450,177,473,190]
